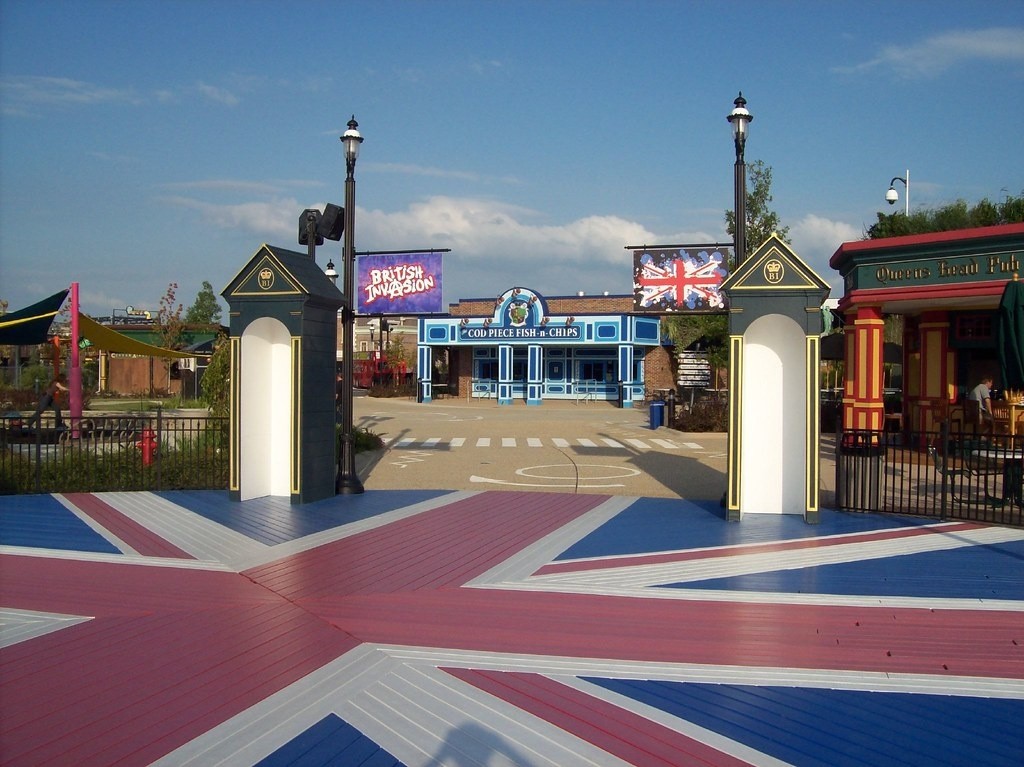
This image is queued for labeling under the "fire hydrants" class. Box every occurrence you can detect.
[135,427,157,469]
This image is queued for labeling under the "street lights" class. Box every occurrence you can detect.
[726,88,756,277]
[334,115,366,494]
[884,170,913,218]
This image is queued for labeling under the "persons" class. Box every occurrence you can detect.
[26,373,70,429]
[970,376,997,443]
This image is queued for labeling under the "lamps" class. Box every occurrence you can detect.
[459,317,469,328]
[540,316,549,326]
[576,291,585,296]
[602,291,610,295]
[496,294,504,306]
[529,293,537,306]
[511,286,520,297]
[564,316,575,326]
[483,317,492,328]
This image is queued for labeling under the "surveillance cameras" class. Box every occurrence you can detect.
[886,188,898,205]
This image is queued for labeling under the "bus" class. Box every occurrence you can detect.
[353,358,393,389]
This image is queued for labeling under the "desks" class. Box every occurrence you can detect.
[703,389,728,408]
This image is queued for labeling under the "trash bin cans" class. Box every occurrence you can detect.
[1002,458,1024,505]
[650,400,665,430]
[834,432,886,513]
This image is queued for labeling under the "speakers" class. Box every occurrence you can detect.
[316,202,346,241]
[298,208,323,246]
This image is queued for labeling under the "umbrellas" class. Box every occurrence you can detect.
[997,281,1024,450]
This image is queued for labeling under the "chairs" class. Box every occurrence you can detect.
[961,400,991,450]
[929,392,960,449]
[990,401,1011,444]
